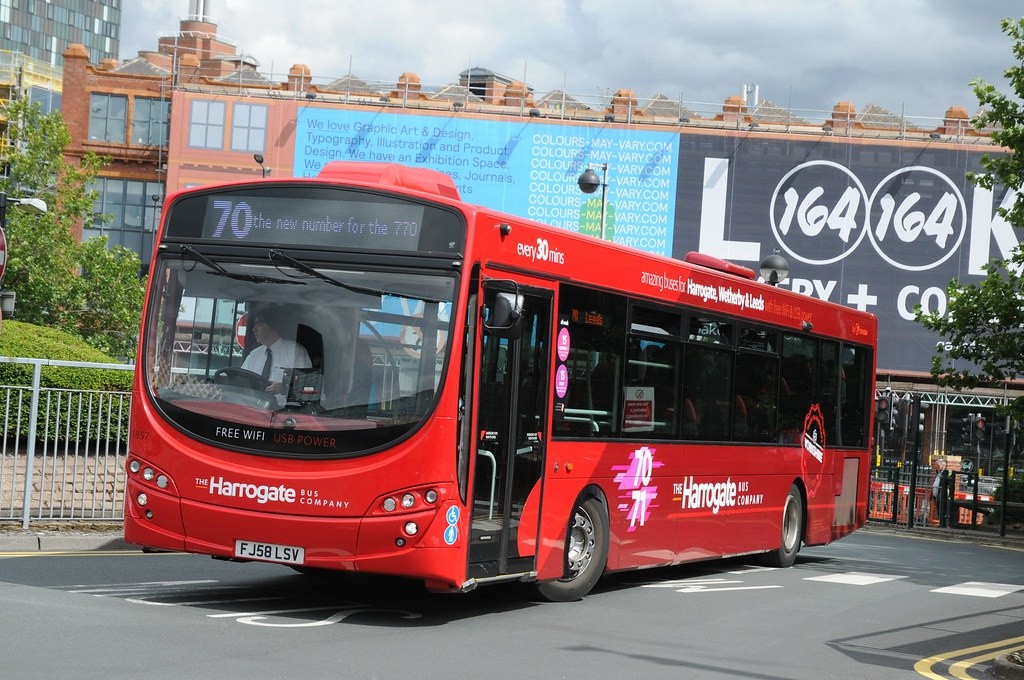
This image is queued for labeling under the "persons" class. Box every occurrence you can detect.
[240,310,313,406]
[931,459,949,526]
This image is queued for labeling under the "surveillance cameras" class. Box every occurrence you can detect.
[577,169,600,193]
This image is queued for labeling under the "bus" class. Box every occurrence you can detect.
[121,159,879,604]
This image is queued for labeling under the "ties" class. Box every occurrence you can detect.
[261,348,272,380]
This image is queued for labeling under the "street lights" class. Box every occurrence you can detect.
[1,190,48,229]
[575,161,610,243]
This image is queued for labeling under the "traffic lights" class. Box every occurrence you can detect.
[973,415,986,443]
[964,416,973,445]
[960,417,968,442]
[912,397,929,435]
[877,396,890,425]
[890,393,901,431]
[999,410,1010,434]
